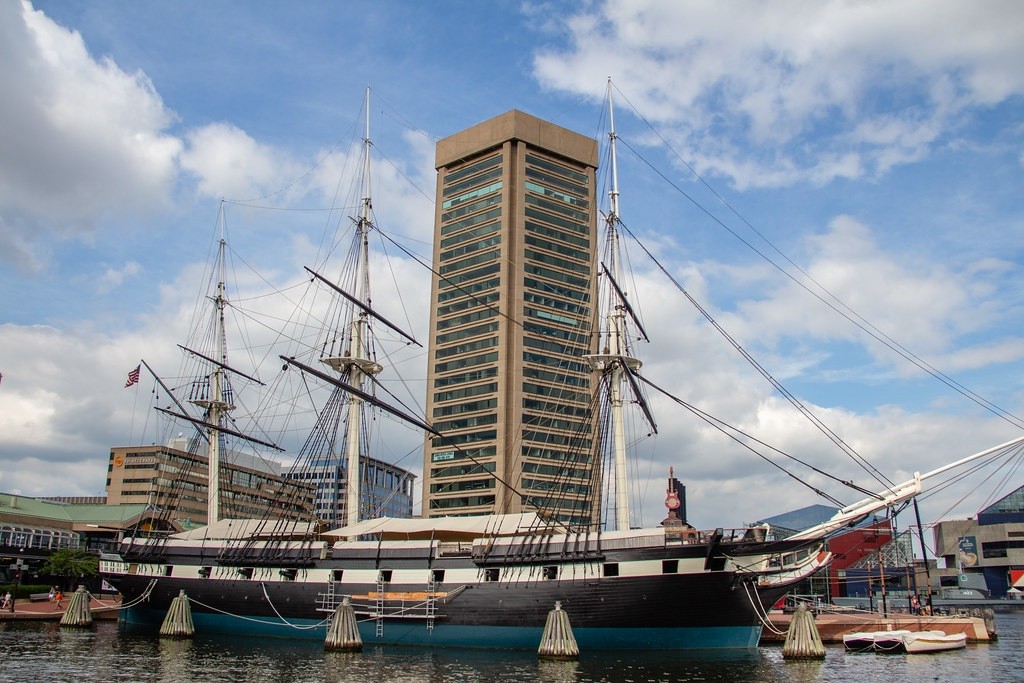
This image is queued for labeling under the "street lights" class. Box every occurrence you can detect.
[9,548,24,613]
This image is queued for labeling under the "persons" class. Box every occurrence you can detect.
[49,587,56,602]
[1,592,11,609]
[924,595,931,615]
[912,595,917,615]
[54,592,63,610]
[915,601,922,616]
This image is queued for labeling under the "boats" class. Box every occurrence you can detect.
[842,624,967,654]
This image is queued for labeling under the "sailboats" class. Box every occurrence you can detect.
[87,74,1024,660]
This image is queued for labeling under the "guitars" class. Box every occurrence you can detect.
[664,465,681,509]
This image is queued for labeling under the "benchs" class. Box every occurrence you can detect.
[783,607,798,614]
[30,593,50,603]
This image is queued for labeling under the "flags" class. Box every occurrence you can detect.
[125,364,140,387]
